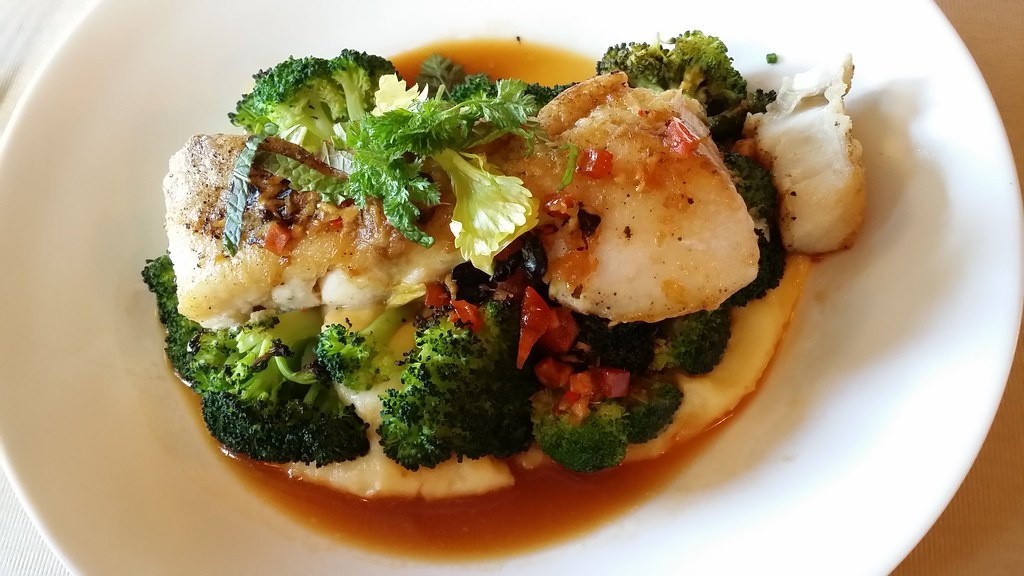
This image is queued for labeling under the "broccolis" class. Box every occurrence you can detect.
[142,33,787,472]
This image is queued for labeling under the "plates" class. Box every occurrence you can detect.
[1,0,1024,576]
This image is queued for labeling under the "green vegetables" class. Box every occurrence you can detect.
[333,78,578,248]
